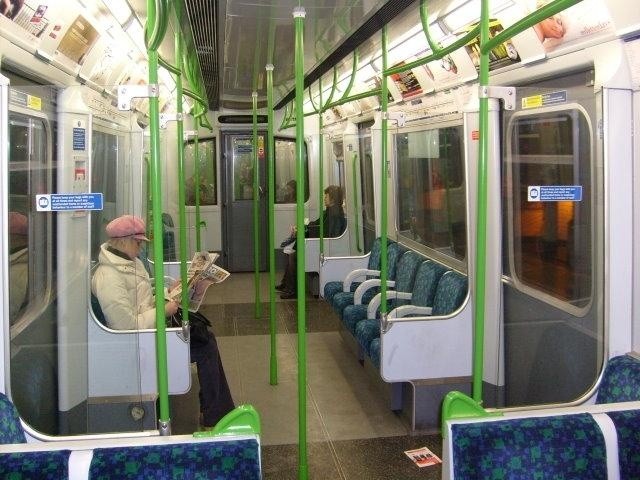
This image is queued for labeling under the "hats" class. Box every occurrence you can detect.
[327,185,343,201]
[106,215,150,242]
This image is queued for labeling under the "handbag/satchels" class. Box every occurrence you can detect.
[171,307,212,346]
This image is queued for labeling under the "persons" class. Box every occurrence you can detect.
[184,167,299,205]
[440,57,458,75]
[275,185,346,299]
[473,42,483,53]
[530,0,565,46]
[8,213,31,327]
[469,44,481,67]
[91,214,235,427]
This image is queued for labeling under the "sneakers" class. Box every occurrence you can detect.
[275,284,286,291]
[281,290,297,300]
